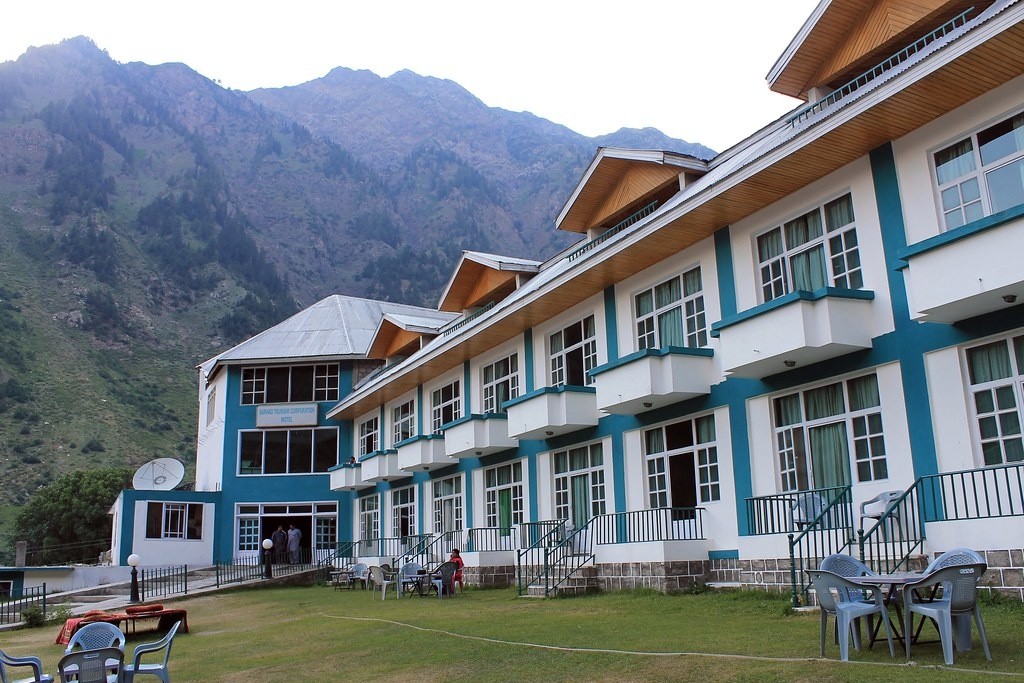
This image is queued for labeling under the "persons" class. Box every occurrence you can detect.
[272,526,287,565]
[445,549,463,594]
[287,524,302,565]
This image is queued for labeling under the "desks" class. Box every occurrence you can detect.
[844,572,943,655]
[330,571,358,592]
[57,658,126,682]
[401,574,442,598]
[56,609,189,645]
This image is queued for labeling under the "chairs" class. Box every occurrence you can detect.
[0,621,183,683]
[348,561,459,601]
[860,489,906,542]
[787,493,830,531]
[807,548,994,663]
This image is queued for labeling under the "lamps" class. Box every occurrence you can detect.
[126,555,142,568]
[260,539,274,550]
